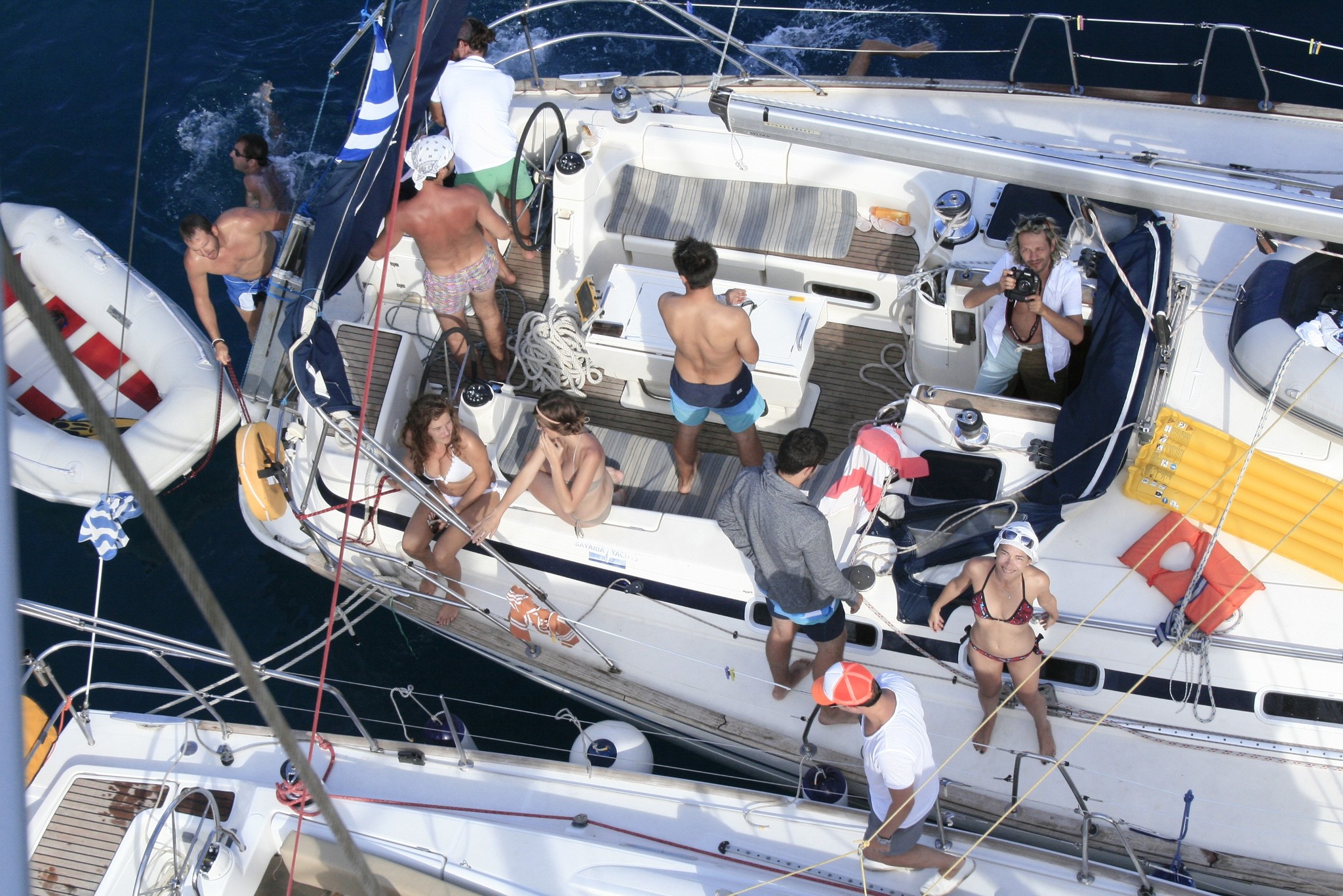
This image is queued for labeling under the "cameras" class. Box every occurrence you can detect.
[1005,267,1039,303]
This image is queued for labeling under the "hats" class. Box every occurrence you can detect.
[812,661,874,707]
[993,521,1040,563]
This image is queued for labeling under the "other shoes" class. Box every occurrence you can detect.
[920,857,976,896]
[863,858,923,871]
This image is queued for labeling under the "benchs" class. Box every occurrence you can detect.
[606,187,921,321]
[497,407,841,534]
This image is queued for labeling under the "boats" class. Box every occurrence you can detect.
[25,609,1205,893]
[229,2,1343,896]
[5,208,242,517]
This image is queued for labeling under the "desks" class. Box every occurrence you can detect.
[585,263,826,436]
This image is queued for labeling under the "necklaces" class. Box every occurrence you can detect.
[993,568,1021,599]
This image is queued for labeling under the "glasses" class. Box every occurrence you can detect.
[1016,217,1051,231]
[534,417,546,429]
[740,300,755,316]
[233,149,250,159]
[429,514,440,534]
[1000,528,1035,550]
[829,703,845,709]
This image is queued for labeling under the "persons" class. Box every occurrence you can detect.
[929,521,1056,765]
[659,238,767,493]
[963,212,1084,409]
[180,18,626,624]
[717,427,864,725]
[810,661,976,896]
[844,38,937,75]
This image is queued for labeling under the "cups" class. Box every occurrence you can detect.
[578,145,595,164]
[581,123,598,146]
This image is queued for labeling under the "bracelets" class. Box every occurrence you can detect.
[213,340,225,348]
[212,338,224,347]
